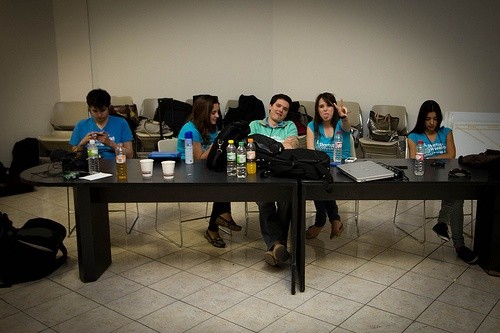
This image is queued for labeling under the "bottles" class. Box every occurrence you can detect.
[236,142,248,179]
[227,140,237,176]
[332,130,343,163]
[88,139,100,174]
[184,131,194,165]
[245,138,257,174]
[114,143,128,179]
[414,140,424,176]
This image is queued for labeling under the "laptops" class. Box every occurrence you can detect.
[337,161,395,182]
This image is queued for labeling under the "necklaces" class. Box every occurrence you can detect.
[425,128,444,155]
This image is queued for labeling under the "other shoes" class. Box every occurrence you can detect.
[305,225,323,239]
[204,230,225,247]
[215,216,242,231]
[274,244,290,262]
[264,251,278,266]
[329,220,344,240]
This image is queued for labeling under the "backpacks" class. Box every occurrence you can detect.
[206,121,251,173]
[0,137,44,197]
[0,212,68,288]
[238,133,285,169]
[261,148,333,184]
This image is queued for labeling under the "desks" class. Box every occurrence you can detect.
[20,157,500,295]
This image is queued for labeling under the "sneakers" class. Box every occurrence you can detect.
[432,222,450,242]
[457,247,478,263]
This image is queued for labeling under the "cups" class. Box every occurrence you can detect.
[161,160,176,179]
[139,159,154,177]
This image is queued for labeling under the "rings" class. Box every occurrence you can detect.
[295,144,297,146]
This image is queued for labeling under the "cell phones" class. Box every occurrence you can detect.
[345,157,357,162]
[93,133,103,136]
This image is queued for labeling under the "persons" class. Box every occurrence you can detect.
[69,88,133,160]
[407,100,477,263]
[306,92,351,241]
[249,94,299,266]
[176,95,242,248]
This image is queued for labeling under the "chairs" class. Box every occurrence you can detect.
[38,96,474,248]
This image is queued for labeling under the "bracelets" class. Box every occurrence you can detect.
[340,116,347,119]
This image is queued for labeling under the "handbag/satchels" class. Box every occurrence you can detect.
[109,97,193,140]
[458,149,500,172]
[366,110,399,142]
[291,105,313,136]
